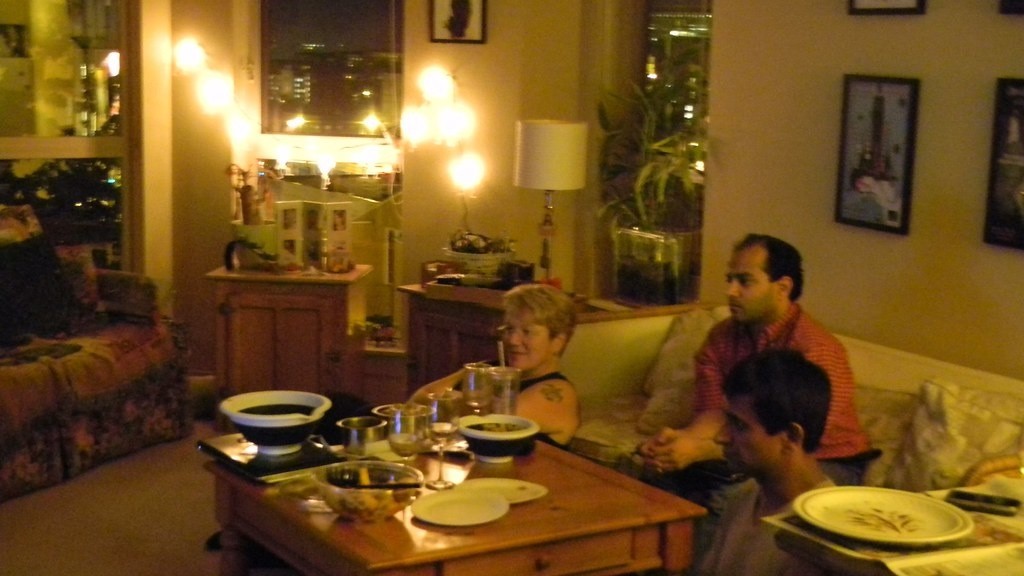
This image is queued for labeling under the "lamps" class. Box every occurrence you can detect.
[512,119,589,279]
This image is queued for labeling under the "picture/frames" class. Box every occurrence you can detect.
[847,0,927,16]
[834,73,920,237]
[427,0,488,44]
[983,77,1024,249]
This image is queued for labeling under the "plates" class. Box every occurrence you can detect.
[791,485,974,545]
[454,478,547,503]
[410,489,508,526]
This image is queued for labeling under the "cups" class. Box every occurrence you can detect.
[490,367,520,415]
[337,418,387,457]
[462,365,492,417]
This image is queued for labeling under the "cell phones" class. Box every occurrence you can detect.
[946,490,1020,517]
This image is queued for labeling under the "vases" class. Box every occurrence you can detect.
[69,34,98,137]
[232,219,278,270]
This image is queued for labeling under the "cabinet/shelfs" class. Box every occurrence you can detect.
[205,261,374,432]
[397,284,600,401]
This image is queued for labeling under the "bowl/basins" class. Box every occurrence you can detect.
[456,415,540,463]
[312,461,424,522]
[219,391,331,453]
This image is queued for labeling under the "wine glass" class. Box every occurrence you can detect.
[425,390,464,490]
[373,405,433,465]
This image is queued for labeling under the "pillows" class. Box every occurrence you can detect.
[637,373,698,434]
[0,233,81,351]
[56,245,107,338]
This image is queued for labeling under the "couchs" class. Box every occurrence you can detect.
[560,305,1024,555]
[0,205,191,504]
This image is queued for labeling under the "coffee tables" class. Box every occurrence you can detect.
[203,432,709,575]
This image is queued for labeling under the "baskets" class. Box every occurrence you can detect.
[444,246,515,285]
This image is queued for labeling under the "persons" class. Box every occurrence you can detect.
[696,347,839,576]
[334,211,345,231]
[403,282,582,452]
[631,232,874,534]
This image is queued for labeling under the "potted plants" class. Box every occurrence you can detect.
[595,58,708,310]
